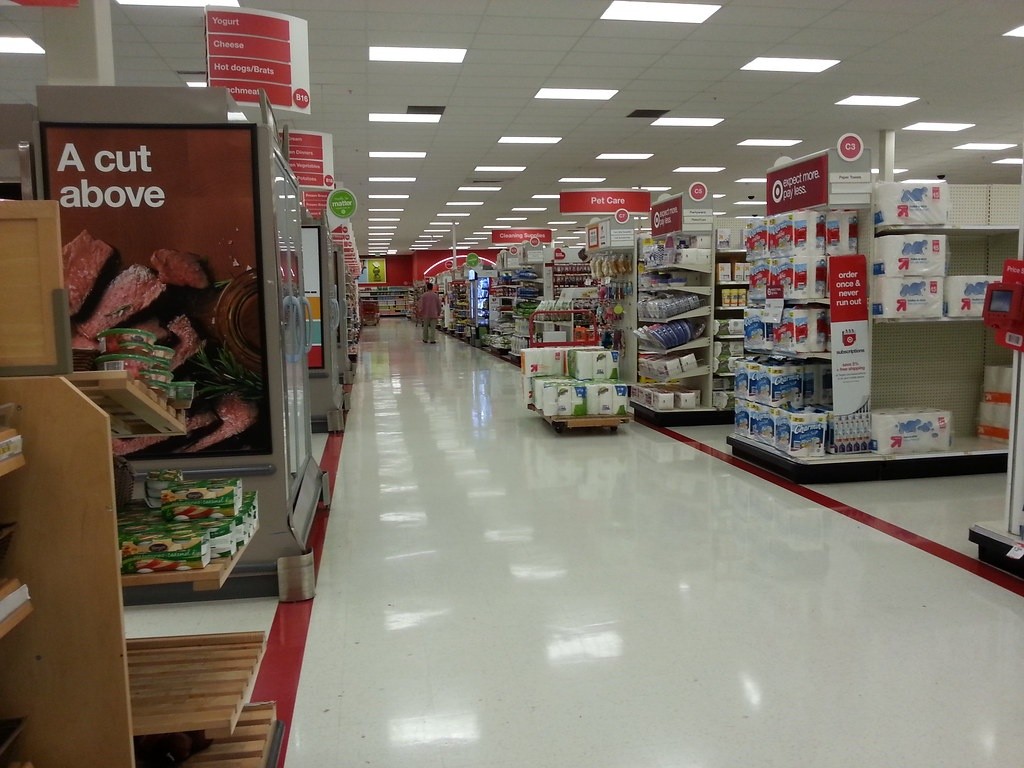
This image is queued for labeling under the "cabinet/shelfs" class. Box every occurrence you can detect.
[728,215,1024,486]
[712,217,750,411]
[346,279,361,362]
[630,263,712,413]
[435,278,544,365]
[358,288,422,319]
[0,368,284,768]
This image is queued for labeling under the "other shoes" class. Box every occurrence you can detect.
[430,340,436,344]
[422,339,428,343]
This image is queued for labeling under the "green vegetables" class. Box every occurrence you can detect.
[188,345,255,401]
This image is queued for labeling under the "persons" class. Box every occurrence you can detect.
[418,280,442,343]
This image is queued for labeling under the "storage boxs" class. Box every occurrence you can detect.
[716,229,731,250]
[740,229,749,249]
[715,263,750,281]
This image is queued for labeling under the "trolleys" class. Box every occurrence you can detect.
[529,308,621,433]
